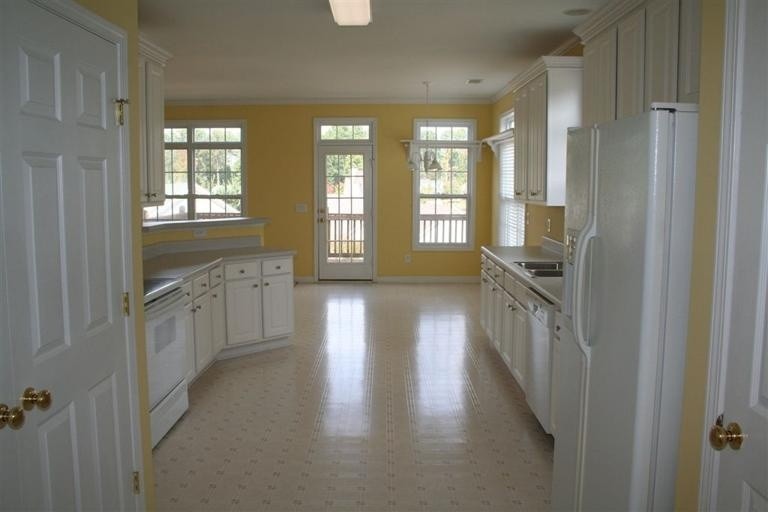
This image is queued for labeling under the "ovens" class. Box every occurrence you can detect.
[145,289,191,411]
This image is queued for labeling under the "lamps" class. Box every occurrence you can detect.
[327,2,373,26]
[403,80,444,178]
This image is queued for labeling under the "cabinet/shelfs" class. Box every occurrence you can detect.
[225,255,295,350]
[572,0,702,126]
[184,261,225,388]
[508,55,581,207]
[479,255,503,353]
[136,35,175,207]
[503,270,531,390]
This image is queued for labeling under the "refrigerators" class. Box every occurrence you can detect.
[550,101,698,512]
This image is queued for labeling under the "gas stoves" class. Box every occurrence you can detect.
[144,275,181,304]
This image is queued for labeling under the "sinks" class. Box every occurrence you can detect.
[527,267,563,278]
[512,259,563,270]
